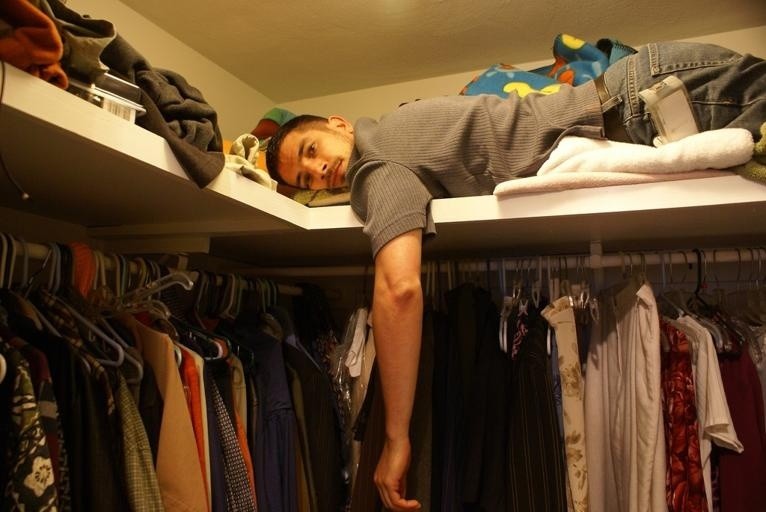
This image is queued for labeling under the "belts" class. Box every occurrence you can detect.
[594,75,632,145]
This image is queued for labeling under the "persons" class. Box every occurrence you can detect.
[262,40,766,511]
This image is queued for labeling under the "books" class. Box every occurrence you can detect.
[65,73,147,124]
